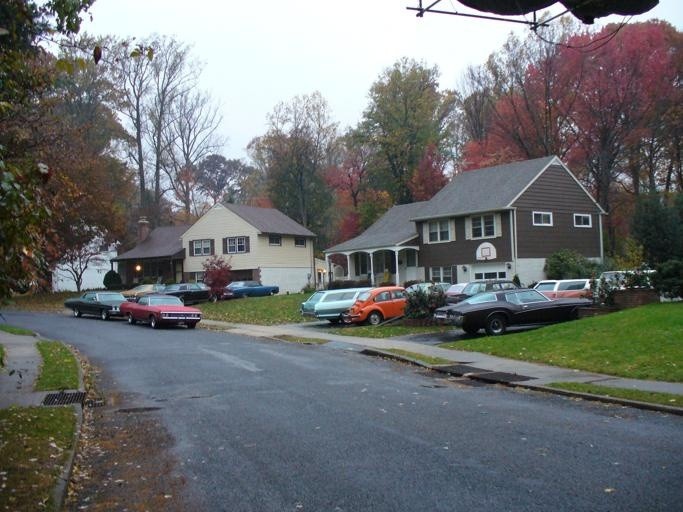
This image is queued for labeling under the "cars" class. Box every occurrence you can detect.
[598,268,657,291]
[13,276,50,292]
[118,294,202,330]
[433,289,595,336]
[404,282,452,297]
[459,278,521,302]
[120,280,280,305]
[340,287,411,325]
[532,279,600,301]
[299,287,390,324]
[444,282,467,306]
[63,291,128,320]
